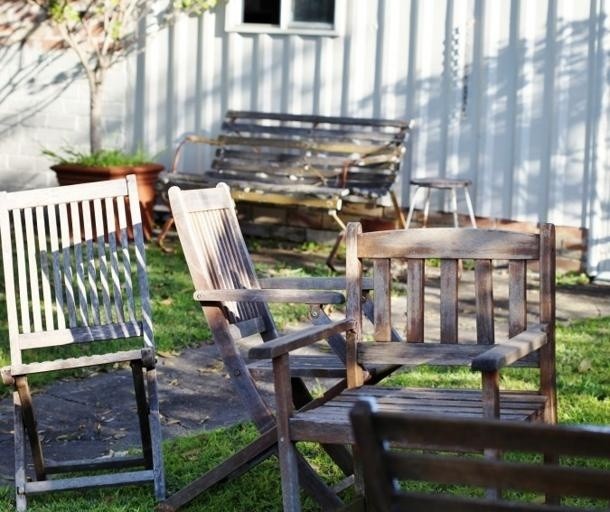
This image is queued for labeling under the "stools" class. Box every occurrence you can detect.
[403,174,483,281]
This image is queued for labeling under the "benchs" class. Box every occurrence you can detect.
[149,114,411,276]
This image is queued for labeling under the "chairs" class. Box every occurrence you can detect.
[159,184,403,509]
[2,174,168,509]
[341,395,607,512]
[247,218,560,511]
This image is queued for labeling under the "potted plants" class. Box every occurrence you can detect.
[35,1,222,241]
